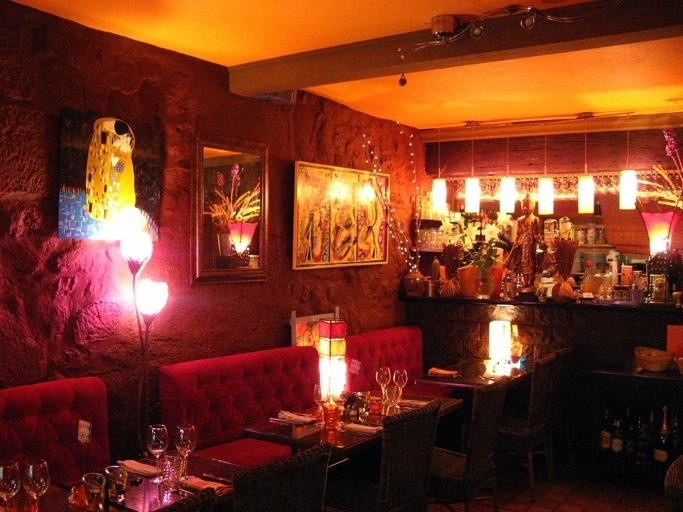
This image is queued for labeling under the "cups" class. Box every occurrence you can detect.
[425,280,440,299]
[81,466,127,511]
[621,266,633,287]
[632,271,642,285]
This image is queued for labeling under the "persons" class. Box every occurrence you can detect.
[505,194,543,287]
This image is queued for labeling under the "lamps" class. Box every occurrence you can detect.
[431,112,640,219]
[228,219,258,256]
[119,230,170,465]
[486,318,513,374]
[318,317,349,430]
[639,210,677,260]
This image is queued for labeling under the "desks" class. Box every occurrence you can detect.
[240,388,464,456]
[415,352,557,393]
[592,366,683,504]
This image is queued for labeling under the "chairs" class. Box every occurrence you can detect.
[431,378,505,510]
[499,352,564,502]
[330,401,441,510]
[158,442,330,512]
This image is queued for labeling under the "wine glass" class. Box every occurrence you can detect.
[0,455,51,512]
[313,384,331,428]
[146,423,197,488]
[375,365,408,408]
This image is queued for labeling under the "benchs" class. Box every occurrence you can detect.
[344,324,454,399]
[0,345,320,512]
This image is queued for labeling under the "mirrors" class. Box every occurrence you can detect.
[186,130,271,288]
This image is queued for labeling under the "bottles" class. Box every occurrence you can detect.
[431,255,440,279]
[460,206,498,227]
[595,400,683,479]
[404,265,425,298]
[344,403,369,425]
[514,273,525,297]
[580,259,594,292]
[577,226,605,246]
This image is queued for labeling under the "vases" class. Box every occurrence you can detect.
[402,270,426,297]
[216,231,231,258]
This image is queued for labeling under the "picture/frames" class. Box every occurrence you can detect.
[290,159,391,272]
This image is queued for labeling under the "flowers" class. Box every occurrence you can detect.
[203,162,262,233]
[636,126,683,212]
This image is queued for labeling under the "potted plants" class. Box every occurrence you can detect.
[457,231,499,300]
